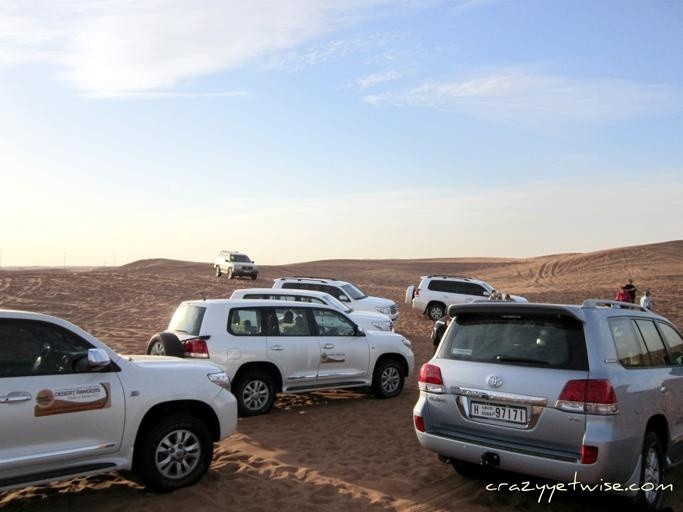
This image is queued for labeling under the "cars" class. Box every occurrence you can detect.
[213,250,259,279]
[0,275,681,512]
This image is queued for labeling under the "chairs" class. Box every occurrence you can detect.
[231,312,251,334]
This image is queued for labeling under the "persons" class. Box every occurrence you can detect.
[616,278,652,312]
[431,315,452,355]
[488,290,510,301]
